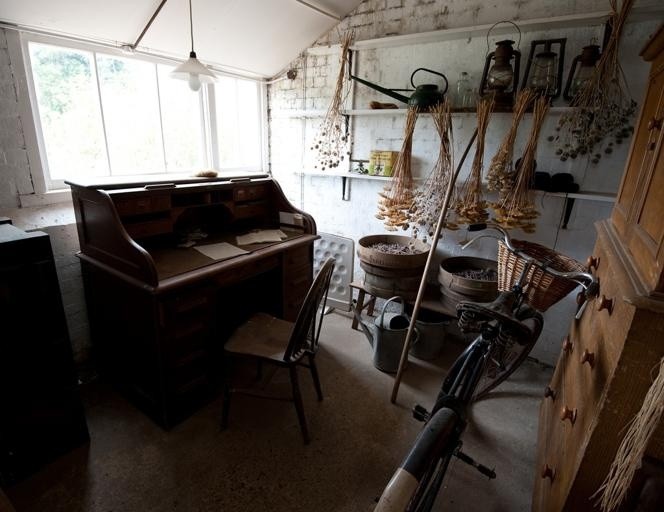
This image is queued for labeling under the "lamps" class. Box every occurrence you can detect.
[479,20,521,113]
[564,45,604,107]
[520,37,566,108]
[170,0,217,91]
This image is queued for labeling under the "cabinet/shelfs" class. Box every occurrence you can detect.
[532,21,664,512]
[289,6,663,228]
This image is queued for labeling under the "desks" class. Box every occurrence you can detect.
[347,277,505,377]
[72,177,321,432]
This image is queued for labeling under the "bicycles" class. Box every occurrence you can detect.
[373,221,598,512]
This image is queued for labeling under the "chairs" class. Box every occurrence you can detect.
[220,259,335,445]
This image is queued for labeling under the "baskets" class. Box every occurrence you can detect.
[499,240,591,312]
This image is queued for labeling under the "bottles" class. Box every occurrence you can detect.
[455,69,474,107]
